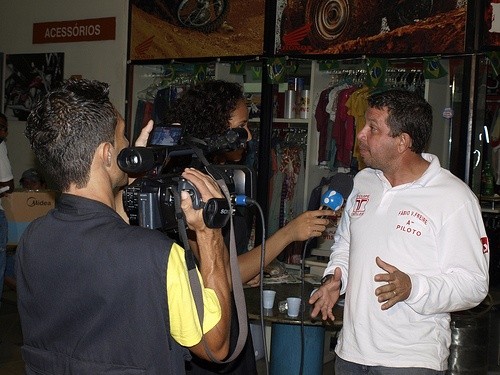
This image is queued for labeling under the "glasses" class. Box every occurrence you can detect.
[22,183,42,190]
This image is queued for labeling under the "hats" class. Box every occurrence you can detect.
[19,168,42,184]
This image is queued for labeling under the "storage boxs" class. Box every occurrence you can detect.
[1,190,55,245]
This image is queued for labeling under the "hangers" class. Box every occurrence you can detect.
[249,127,308,144]
[331,68,426,91]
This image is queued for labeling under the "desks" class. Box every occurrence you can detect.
[244,275,344,375]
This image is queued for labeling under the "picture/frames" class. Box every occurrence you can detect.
[268,0,476,56]
[126,0,268,59]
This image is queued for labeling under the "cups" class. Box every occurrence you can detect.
[263,290,276,308]
[287,298,301,317]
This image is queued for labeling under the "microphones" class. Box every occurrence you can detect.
[308,173,355,241]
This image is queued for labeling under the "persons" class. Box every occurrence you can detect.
[308,89,491,375]
[139,81,352,375]
[16,73,249,374]
